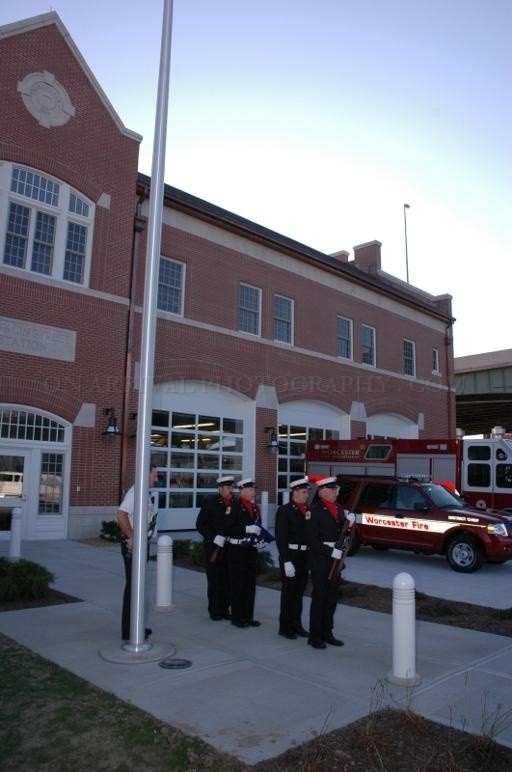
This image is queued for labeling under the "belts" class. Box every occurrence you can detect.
[323,541,336,548]
[226,536,257,545]
[288,543,309,551]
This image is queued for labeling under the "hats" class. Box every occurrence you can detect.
[316,477,340,489]
[216,476,234,487]
[289,478,312,491]
[237,478,256,490]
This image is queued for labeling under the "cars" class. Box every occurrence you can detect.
[0,471,61,504]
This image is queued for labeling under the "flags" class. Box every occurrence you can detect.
[405,204,410,208]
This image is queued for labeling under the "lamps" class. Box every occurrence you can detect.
[101,407,122,437]
[263,427,281,453]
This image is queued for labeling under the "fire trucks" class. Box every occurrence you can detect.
[305,437,512,516]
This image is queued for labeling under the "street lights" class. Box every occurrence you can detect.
[403,204,410,284]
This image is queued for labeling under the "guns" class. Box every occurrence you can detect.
[327,480,366,581]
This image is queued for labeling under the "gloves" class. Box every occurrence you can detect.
[245,525,262,537]
[331,548,343,560]
[214,535,226,548]
[284,561,297,577]
[347,512,355,528]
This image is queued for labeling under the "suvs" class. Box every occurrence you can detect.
[305,472,512,574]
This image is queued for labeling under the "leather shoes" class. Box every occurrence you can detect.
[279,625,343,648]
[209,611,260,628]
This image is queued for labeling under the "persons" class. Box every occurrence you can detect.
[195,476,236,621]
[274,478,312,640]
[230,477,263,629]
[116,464,159,641]
[304,473,345,649]
